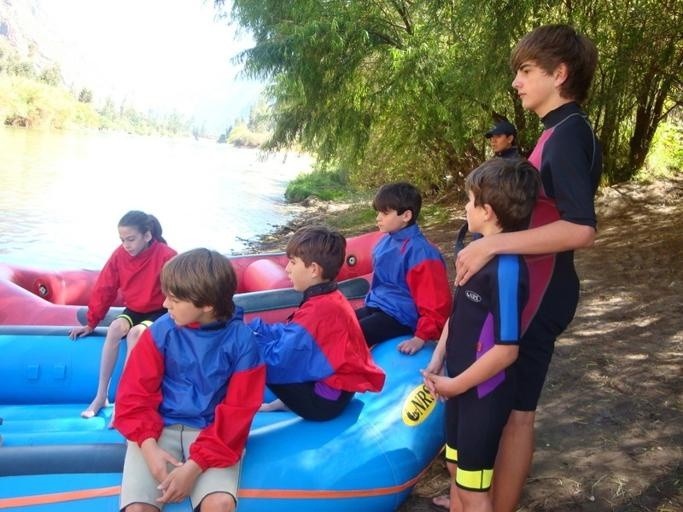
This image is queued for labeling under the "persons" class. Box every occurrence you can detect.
[418,157,542,512]
[112,247,267,512]
[67,210,179,430]
[484,120,523,158]
[257,182,452,412]
[247,225,386,421]
[454,24,602,512]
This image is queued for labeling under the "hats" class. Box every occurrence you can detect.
[486,123,514,138]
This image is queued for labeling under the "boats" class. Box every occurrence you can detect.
[0,221,443,325]
[0,328,451,511]
